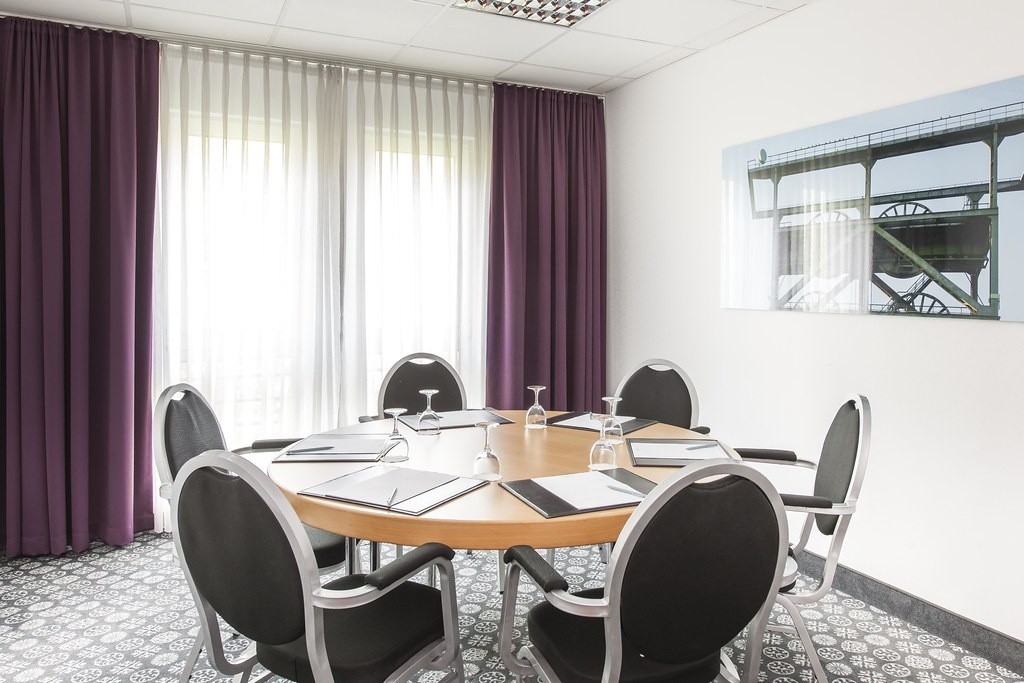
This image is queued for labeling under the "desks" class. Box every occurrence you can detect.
[268,410,743,576]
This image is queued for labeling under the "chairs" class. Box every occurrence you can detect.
[547,358,711,568]
[359,351,505,594]
[733,392,872,683]
[498,459,789,683]
[171,447,465,683]
[150,383,361,683]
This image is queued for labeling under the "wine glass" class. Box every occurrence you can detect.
[383,408,408,458]
[601,397,622,444]
[525,386,546,427]
[475,421,501,480]
[589,416,615,468]
[417,389,439,434]
